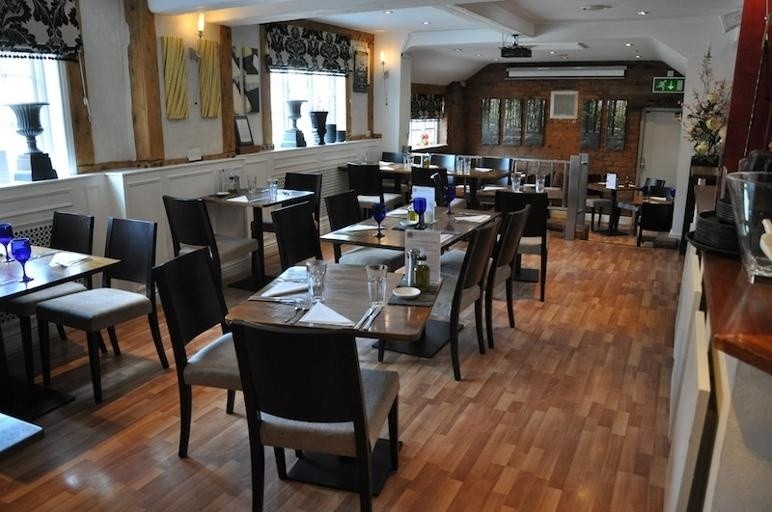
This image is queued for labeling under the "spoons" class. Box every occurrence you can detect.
[284,307,310,324]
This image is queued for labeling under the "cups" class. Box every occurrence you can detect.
[726,171,772,283]
[511,173,522,192]
[365,264,388,307]
[247,175,257,192]
[456,156,463,174]
[267,179,278,201]
[306,259,328,304]
[403,152,411,165]
[536,173,547,193]
[324,124,336,143]
[414,156,421,164]
[464,157,471,174]
[336,130,346,142]
[214,168,226,195]
[360,151,368,165]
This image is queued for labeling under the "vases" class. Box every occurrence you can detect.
[693,140,715,157]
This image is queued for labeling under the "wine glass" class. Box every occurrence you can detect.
[413,198,427,229]
[12,238,34,283]
[0,223,15,262]
[372,204,386,238]
[443,184,456,214]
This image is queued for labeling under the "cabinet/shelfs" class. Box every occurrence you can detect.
[661,183,771,512]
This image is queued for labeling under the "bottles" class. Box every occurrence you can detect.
[408,249,420,288]
[423,157,430,168]
[415,255,430,290]
[228,175,236,196]
[624,176,630,187]
[407,207,418,221]
[616,178,620,187]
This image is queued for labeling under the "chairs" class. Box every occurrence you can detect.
[586,171,677,248]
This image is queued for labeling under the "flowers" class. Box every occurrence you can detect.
[676,45,734,157]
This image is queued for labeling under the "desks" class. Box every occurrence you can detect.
[677,154,721,256]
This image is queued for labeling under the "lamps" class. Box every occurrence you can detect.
[188,11,207,64]
[379,50,389,81]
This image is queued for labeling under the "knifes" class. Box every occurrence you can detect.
[353,306,384,330]
[248,296,304,303]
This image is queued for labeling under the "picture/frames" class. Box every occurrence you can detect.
[232,115,255,147]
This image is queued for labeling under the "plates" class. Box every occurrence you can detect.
[392,286,422,300]
[685,198,741,259]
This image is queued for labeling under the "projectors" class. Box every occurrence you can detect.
[500,46,532,57]
